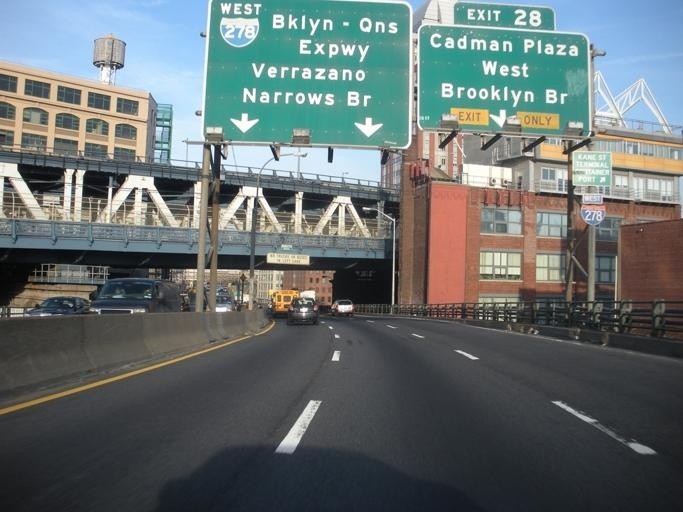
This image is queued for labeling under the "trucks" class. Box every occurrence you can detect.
[271,290,316,313]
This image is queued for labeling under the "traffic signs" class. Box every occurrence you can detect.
[571,150,613,187]
[417,23,592,138]
[202,0,413,150]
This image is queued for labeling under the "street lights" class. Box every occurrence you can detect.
[362,207,396,314]
[248,152,308,310]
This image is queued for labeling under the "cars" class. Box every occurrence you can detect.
[24,278,236,318]
[287,298,320,326]
[331,299,355,317]
